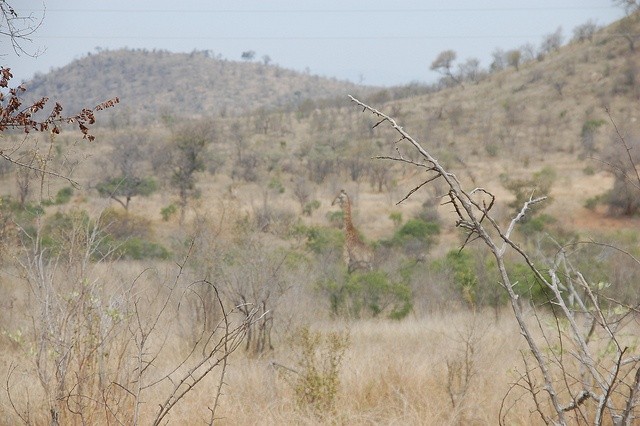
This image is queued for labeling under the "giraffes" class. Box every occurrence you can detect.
[331,188,374,274]
